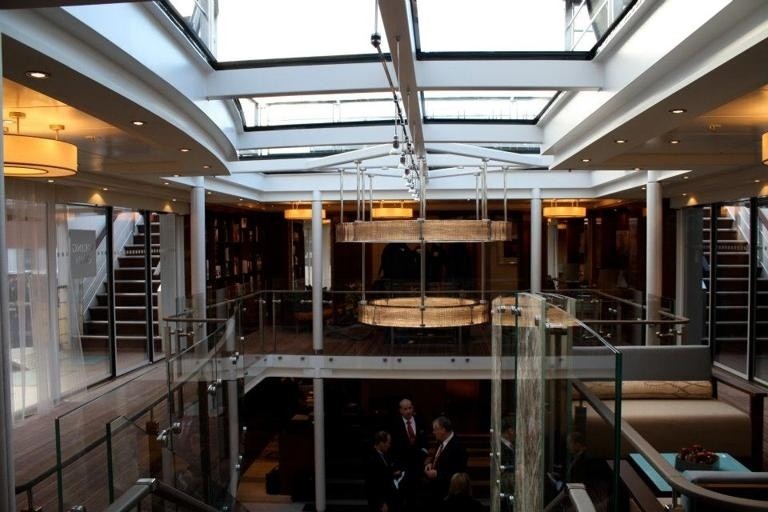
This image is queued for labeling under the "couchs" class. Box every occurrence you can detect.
[568,345,767,464]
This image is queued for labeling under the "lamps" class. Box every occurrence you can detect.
[542,197,587,218]
[284,200,327,219]
[370,1,428,203]
[370,199,413,219]
[0,112,79,178]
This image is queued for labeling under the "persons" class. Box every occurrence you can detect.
[391,396,428,449]
[545,430,599,500]
[424,415,465,488]
[441,470,490,512]
[361,429,405,511]
[501,419,516,474]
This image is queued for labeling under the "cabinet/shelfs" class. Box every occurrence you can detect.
[183,212,263,339]
[264,211,304,325]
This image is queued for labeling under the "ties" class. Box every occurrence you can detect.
[432,443,443,469]
[406,419,416,448]
